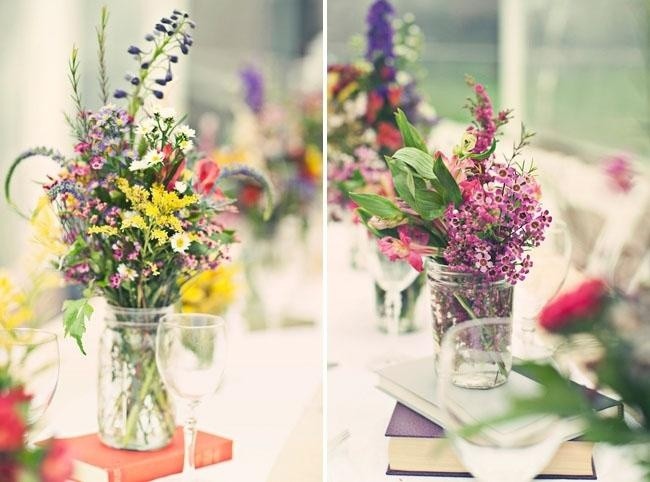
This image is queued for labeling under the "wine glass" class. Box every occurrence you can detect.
[364,234,427,374]
[153,310,228,482]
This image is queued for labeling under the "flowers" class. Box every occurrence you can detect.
[348,75,553,286]
[4,5,241,307]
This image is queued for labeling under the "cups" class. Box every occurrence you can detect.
[0,326,60,451]
[432,315,580,479]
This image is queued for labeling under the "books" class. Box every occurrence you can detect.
[371,350,626,451]
[384,399,600,479]
[47,422,234,482]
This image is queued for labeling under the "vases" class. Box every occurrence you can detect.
[424,258,516,390]
[95,307,180,452]
